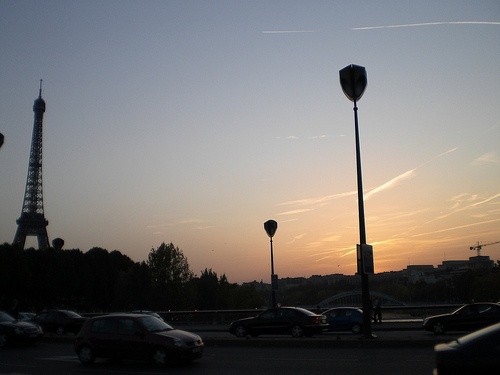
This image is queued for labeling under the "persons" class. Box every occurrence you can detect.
[374,295,382,323]
[7,300,20,320]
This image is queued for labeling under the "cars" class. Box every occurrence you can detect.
[73,310,204,367]
[431,322,499,375]
[29,310,88,336]
[13,313,39,327]
[421,301,500,334]
[228,304,329,338]
[317,306,363,334]
[0,311,44,347]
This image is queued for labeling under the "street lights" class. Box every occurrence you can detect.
[341,64,379,339]
[265,217,279,303]
